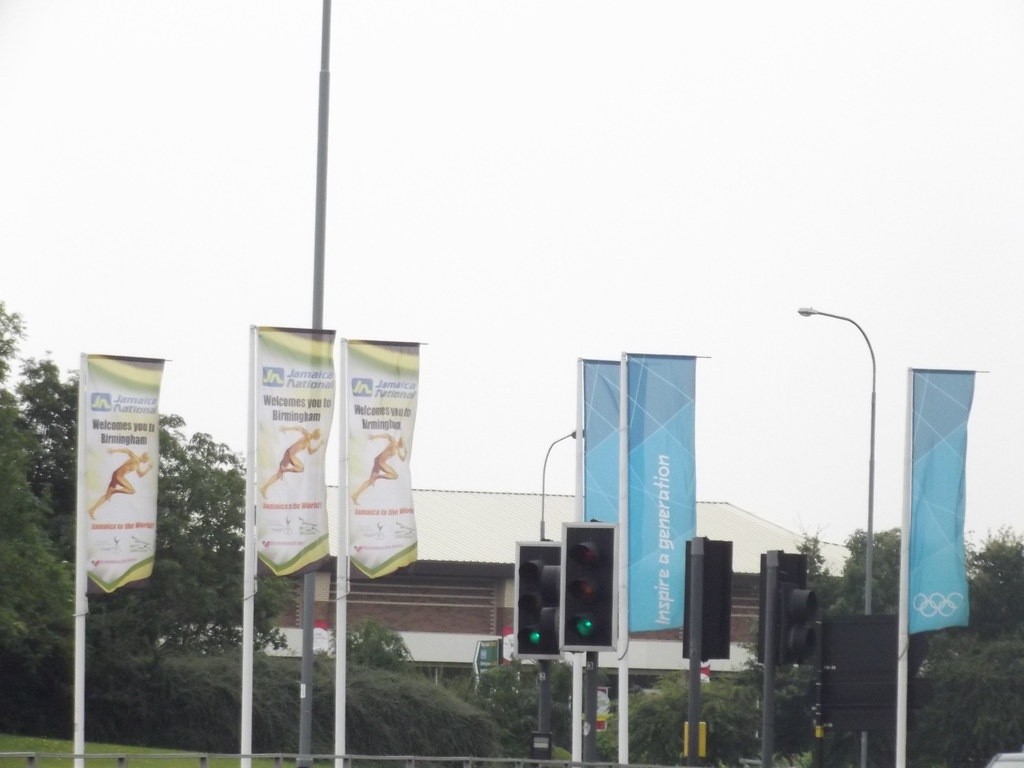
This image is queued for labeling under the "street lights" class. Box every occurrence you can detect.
[799,305,876,768]
[539,429,586,541]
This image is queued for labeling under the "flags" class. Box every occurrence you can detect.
[582,352,699,632]
[907,367,976,635]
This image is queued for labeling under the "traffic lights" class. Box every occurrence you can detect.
[757,551,818,665]
[513,542,566,660]
[559,522,619,651]
